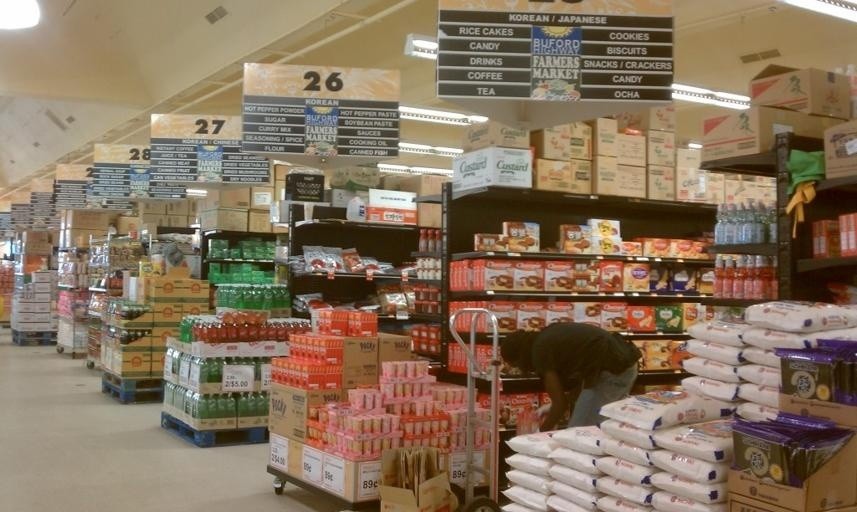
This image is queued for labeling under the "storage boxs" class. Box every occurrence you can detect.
[0,228,58,332]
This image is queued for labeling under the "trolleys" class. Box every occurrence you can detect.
[447,306,502,510]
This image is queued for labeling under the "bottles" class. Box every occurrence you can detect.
[162,283,313,418]
[712,197,779,300]
[408,230,446,357]
[105,299,151,345]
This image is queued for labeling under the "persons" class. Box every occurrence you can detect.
[498,322,642,432]
